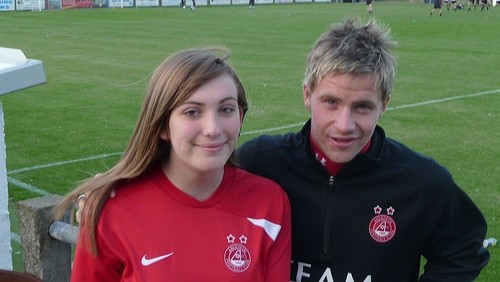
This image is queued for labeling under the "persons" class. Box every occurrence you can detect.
[52,46,292,282]
[176,0,491,17]
[75,16,491,282]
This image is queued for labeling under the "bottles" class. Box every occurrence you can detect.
[483,237,498,249]
